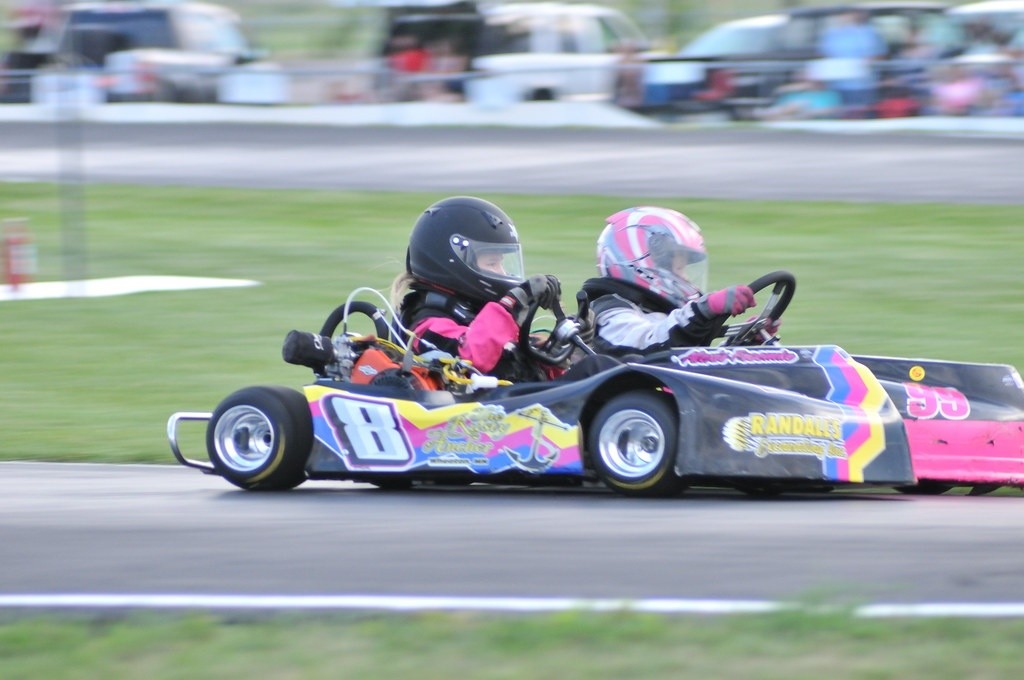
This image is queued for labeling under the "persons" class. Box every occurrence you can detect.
[588,206,780,358]
[786,5,1024,127]
[391,196,594,384]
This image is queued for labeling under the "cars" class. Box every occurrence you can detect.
[52,0,246,101]
[639,0,1024,116]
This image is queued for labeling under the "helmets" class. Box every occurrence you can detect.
[405,196,524,302]
[597,206,707,307]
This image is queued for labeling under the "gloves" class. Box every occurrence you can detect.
[507,274,561,310]
[568,309,596,343]
[697,285,756,320]
[742,315,782,343]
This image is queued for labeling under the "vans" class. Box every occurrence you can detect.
[374,0,650,104]
